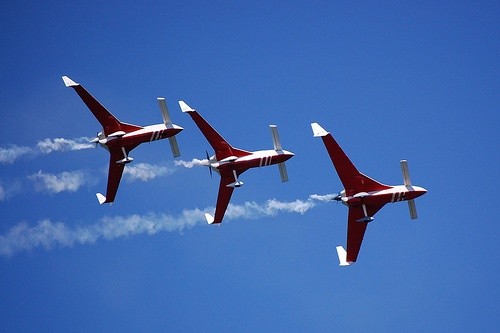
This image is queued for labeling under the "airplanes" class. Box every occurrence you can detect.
[176,97,296,225]
[60,73,186,206]
[310,122,429,267]
